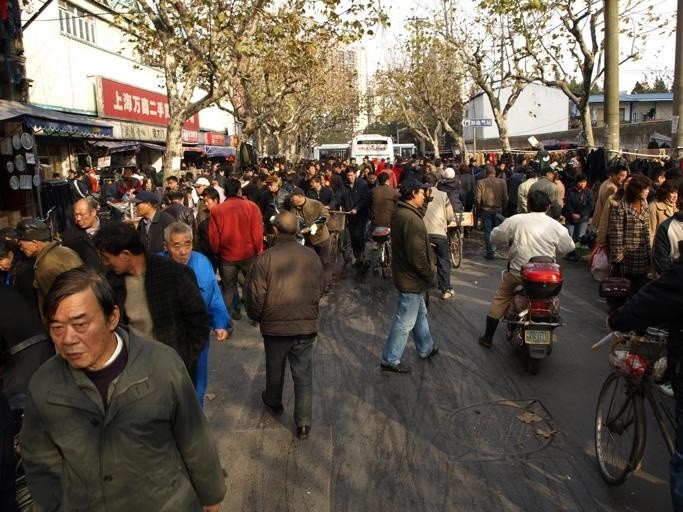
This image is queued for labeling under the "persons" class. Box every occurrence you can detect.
[0,141,683,512]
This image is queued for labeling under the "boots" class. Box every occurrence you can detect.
[478,315,499,348]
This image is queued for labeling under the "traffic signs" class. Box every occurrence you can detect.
[460,118,493,128]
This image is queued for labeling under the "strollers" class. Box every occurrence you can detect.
[106,191,137,222]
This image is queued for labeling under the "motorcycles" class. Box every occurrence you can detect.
[267,201,326,246]
[493,212,565,375]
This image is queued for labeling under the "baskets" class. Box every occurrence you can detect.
[608,326,668,384]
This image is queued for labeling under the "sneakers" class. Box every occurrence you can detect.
[440,289,454,300]
[379,358,411,373]
[483,252,496,260]
[421,345,438,358]
[260,390,284,414]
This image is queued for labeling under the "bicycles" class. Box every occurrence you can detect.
[449,209,464,270]
[590,325,678,488]
[37,205,58,240]
[323,208,353,265]
[372,227,391,280]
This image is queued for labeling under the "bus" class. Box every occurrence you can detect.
[345,133,396,166]
[311,141,418,163]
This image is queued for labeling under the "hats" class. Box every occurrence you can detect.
[5,216,51,241]
[286,187,304,199]
[398,177,431,192]
[264,176,278,183]
[129,189,158,205]
[190,177,210,187]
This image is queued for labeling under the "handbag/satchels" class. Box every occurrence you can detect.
[598,276,631,299]
